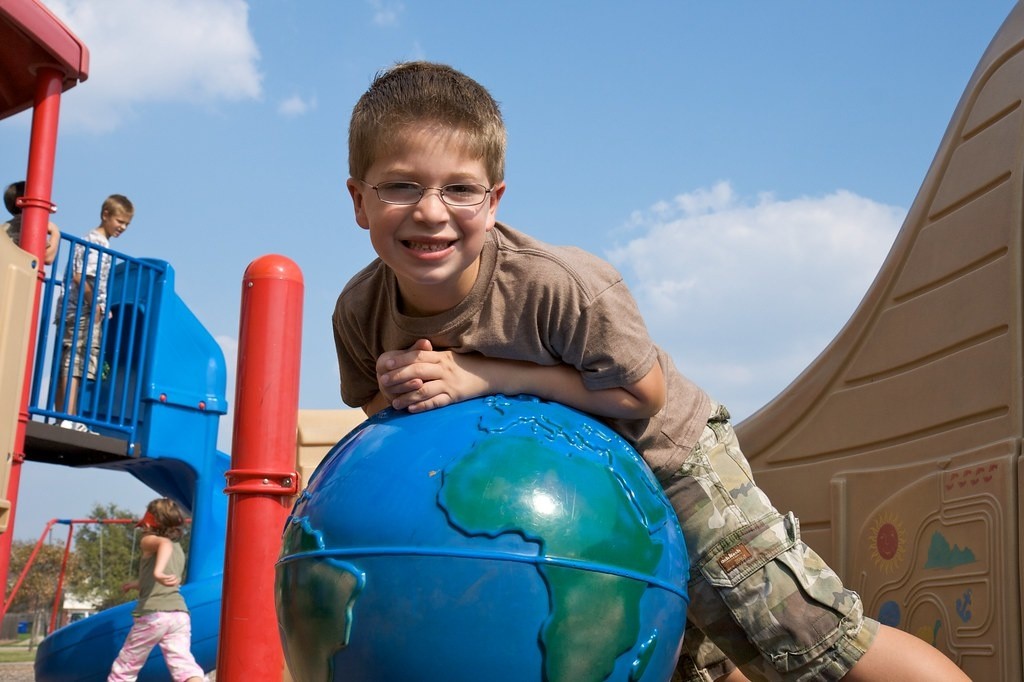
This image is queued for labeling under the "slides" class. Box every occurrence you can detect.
[33,450,233,682]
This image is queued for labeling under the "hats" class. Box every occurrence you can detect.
[133,511,163,529]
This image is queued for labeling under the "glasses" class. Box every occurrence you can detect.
[350,169,498,208]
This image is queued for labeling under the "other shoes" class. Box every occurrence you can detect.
[60,418,100,436]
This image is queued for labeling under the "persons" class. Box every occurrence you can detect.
[331,61,973,682]
[2,181,62,266]
[105,498,205,681]
[52,195,135,435]
